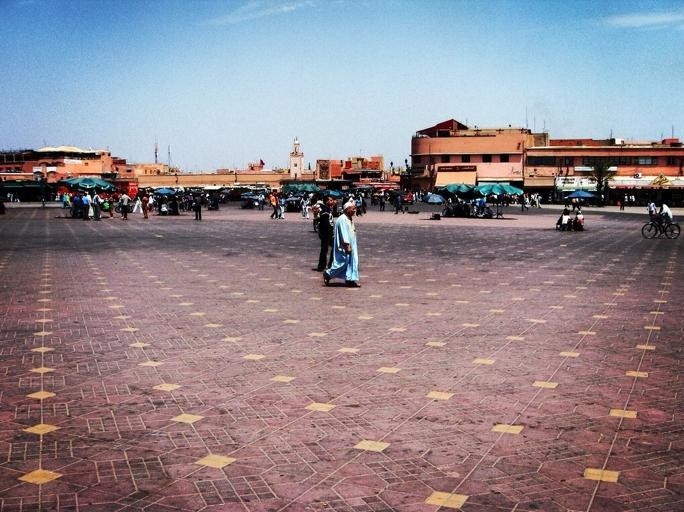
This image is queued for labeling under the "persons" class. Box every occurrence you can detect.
[490,192,542,212]
[63,189,153,222]
[322,200,361,287]
[442,200,494,218]
[344,188,406,214]
[158,189,310,220]
[42,191,60,208]
[598,192,677,211]
[556,197,585,232]
[318,197,340,269]
[7,191,20,202]
[646,200,673,223]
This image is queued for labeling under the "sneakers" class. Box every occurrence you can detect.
[323,272,330,286]
[346,280,361,288]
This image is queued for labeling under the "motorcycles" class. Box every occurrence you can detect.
[114,202,132,214]
[313,215,335,232]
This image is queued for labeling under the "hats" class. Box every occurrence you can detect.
[343,202,352,212]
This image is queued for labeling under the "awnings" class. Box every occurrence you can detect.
[607,181,684,189]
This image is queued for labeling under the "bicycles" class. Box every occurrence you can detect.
[641,214,680,240]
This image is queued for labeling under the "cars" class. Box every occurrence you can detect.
[389,191,413,205]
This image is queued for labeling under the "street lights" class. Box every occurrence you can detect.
[418,134,433,195]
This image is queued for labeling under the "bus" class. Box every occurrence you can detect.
[55,178,138,201]
